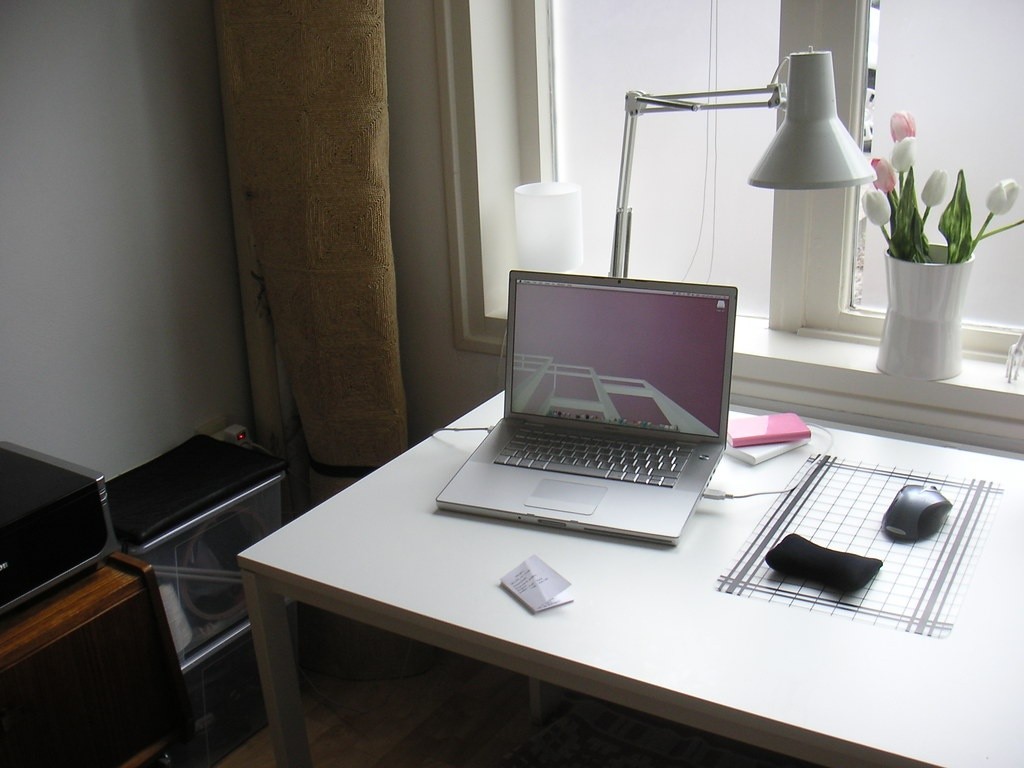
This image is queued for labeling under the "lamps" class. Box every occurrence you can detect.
[608,45,878,280]
[515,180,584,276]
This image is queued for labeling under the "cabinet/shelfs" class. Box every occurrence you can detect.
[0,552,196,768]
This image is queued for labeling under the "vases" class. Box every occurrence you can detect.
[878,242,976,378]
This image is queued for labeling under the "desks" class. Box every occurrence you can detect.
[235,388,1024,768]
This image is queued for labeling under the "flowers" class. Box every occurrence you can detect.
[861,111,1021,264]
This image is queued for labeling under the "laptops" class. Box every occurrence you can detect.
[436,270,737,545]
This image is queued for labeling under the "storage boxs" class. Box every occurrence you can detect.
[120,436,306,768]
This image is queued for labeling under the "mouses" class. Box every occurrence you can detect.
[882,485,954,543]
